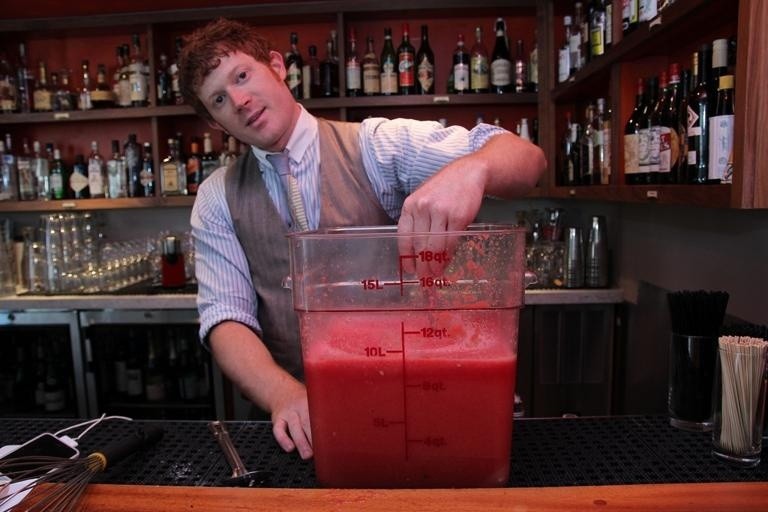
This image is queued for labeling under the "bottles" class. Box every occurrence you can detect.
[157,31,339,105]
[563,228,585,288]
[162,236,185,290]
[12,328,211,413]
[159,132,250,195]
[556,38,736,187]
[0,134,155,202]
[558,0,657,83]
[586,217,609,290]
[0,35,149,113]
[346,19,538,97]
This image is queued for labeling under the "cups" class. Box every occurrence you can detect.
[523,243,563,287]
[37,212,156,295]
[668,333,719,434]
[712,349,768,469]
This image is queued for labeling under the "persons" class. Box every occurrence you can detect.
[177,18,546,463]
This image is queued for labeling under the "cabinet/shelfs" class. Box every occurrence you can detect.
[0,0,767,209]
[1,302,631,417]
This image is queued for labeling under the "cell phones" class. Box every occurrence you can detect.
[0,433,81,484]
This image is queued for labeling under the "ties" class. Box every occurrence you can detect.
[266,151,310,231]
